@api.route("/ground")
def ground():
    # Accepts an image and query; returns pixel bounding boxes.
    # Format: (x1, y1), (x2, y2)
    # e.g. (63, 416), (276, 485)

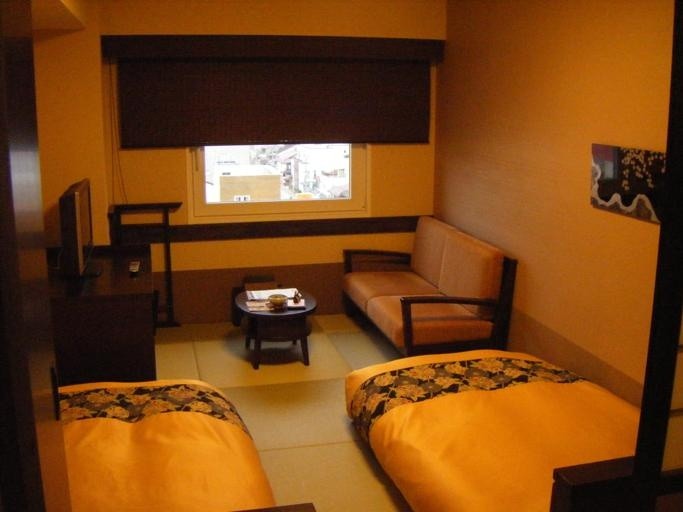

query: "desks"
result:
(55, 244), (159, 383)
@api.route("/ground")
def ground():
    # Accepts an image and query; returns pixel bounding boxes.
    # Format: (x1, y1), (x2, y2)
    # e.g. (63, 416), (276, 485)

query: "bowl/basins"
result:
(268, 294), (288, 308)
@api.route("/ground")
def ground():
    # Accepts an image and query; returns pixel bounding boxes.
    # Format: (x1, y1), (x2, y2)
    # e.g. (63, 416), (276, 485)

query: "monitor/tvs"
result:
(59, 178), (102, 290)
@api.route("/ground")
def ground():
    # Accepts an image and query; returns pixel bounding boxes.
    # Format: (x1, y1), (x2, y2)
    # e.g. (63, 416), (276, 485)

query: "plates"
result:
(286, 297), (307, 309)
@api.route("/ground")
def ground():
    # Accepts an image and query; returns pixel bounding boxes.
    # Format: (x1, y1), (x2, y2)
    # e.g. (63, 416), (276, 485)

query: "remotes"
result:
(128, 260), (141, 272)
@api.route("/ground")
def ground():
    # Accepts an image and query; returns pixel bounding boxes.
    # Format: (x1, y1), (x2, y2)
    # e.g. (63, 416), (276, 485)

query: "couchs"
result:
(340, 211), (517, 354)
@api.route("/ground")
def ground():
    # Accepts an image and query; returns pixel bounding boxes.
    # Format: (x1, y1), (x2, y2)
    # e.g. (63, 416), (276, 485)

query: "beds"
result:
(54, 375), (316, 511)
(343, 346), (637, 511)
